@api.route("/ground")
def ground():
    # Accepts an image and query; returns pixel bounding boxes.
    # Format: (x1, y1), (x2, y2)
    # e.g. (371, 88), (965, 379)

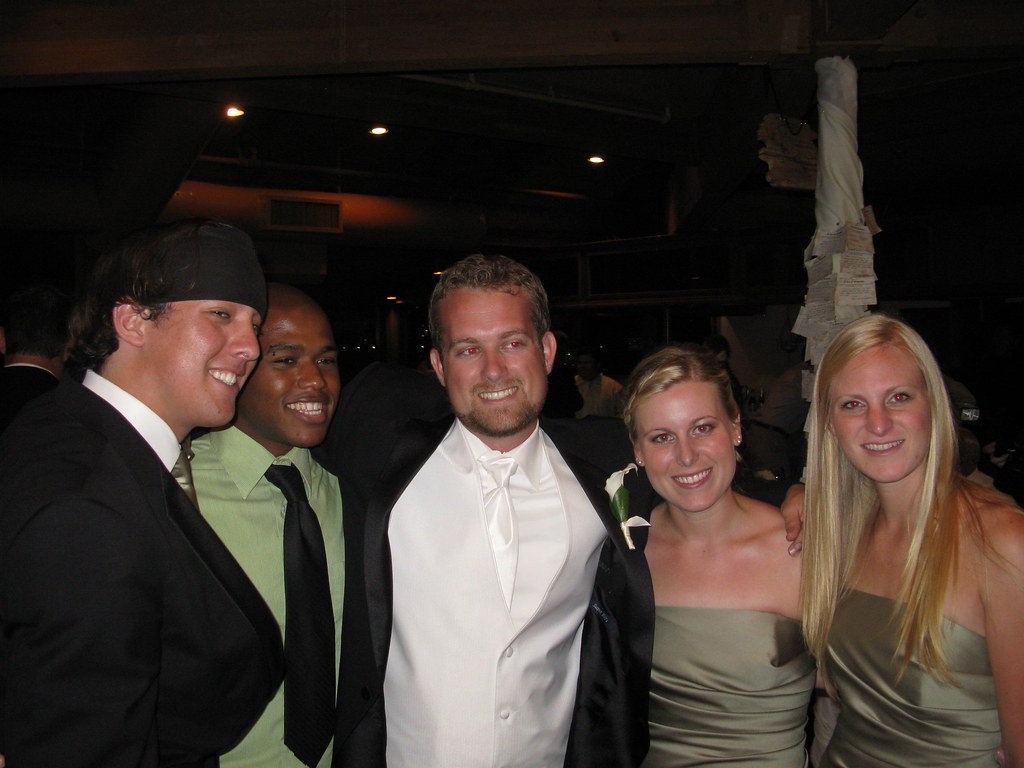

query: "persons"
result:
(189, 283), (345, 768)
(0, 244), (86, 435)
(548, 330), (584, 418)
(960, 424), (1024, 508)
(316, 254), (804, 768)
(803, 313), (1024, 768)
(622, 345), (825, 768)
(0, 222), (286, 768)
(571, 349), (621, 419)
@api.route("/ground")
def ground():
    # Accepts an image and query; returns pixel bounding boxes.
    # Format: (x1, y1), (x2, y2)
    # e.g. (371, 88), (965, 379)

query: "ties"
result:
(480, 457), (519, 607)
(268, 464), (340, 767)
